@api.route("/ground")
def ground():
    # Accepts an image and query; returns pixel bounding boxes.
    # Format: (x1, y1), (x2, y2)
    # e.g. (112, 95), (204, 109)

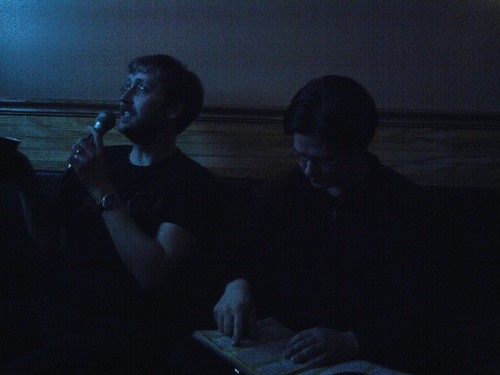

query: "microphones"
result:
(63, 111), (116, 176)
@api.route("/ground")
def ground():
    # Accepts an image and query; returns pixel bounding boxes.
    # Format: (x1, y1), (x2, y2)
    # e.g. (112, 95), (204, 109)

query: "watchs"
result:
(97, 193), (121, 217)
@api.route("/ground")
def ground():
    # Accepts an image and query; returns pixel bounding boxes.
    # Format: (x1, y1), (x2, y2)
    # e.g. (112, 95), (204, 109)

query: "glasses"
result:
(290, 144), (358, 165)
(120, 84), (168, 102)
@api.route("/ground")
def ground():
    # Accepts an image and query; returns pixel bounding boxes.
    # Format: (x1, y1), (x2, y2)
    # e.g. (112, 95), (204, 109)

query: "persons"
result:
(194, 76), (435, 375)
(0, 55), (220, 375)
